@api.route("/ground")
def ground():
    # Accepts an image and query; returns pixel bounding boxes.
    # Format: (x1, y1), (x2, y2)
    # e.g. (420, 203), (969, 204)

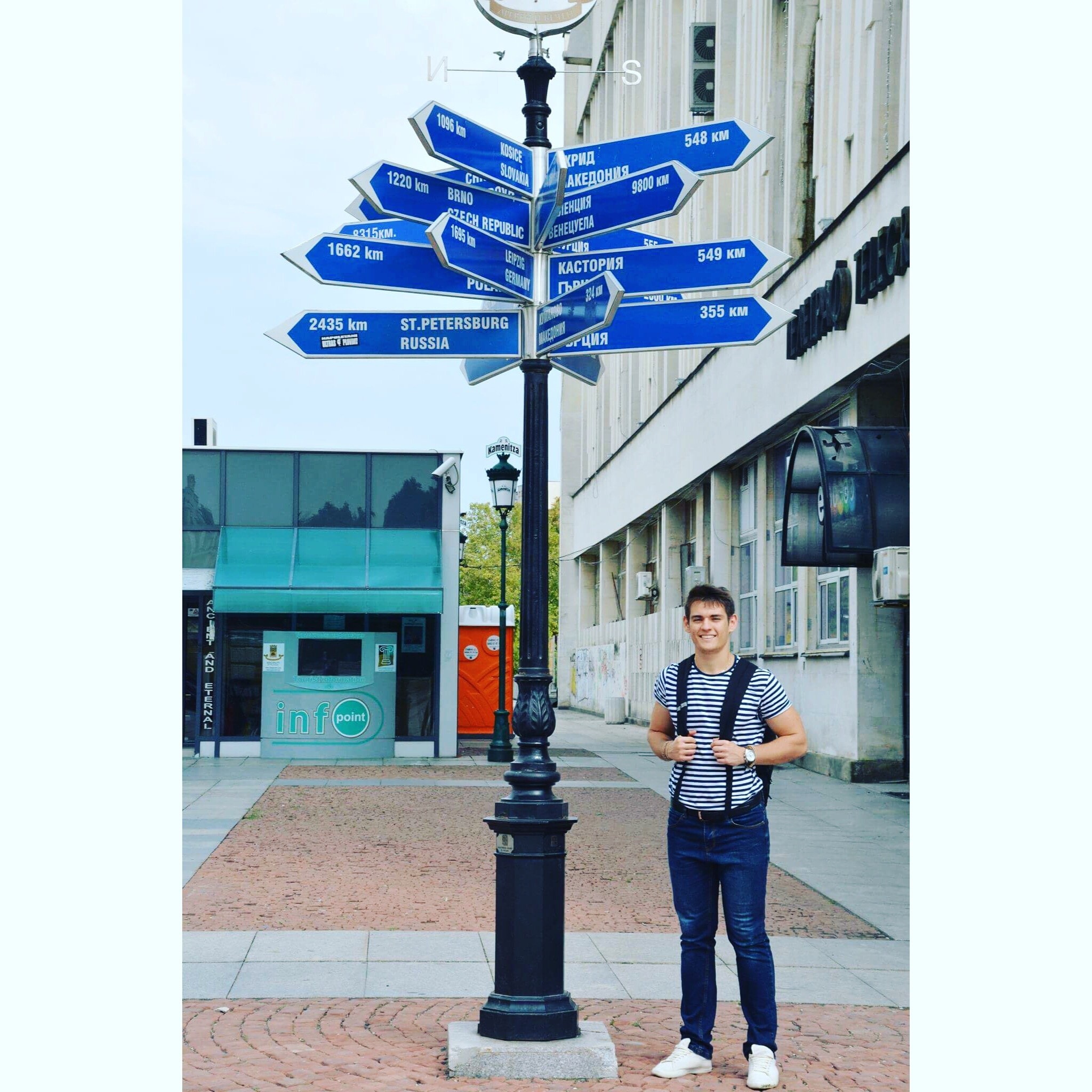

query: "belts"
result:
(670, 792), (762, 822)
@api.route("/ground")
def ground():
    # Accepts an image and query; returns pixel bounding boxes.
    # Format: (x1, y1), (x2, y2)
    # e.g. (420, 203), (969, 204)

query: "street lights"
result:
(485, 437), (521, 762)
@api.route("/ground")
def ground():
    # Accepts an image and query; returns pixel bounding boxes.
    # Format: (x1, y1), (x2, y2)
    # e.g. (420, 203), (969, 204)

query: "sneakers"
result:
(651, 1038), (712, 1079)
(747, 1044), (778, 1088)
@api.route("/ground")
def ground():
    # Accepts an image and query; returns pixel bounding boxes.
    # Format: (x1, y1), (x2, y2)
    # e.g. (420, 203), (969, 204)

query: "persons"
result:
(647, 583), (810, 1091)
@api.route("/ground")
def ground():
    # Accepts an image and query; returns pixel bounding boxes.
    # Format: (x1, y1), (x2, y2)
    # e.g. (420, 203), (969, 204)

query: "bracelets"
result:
(748, 745), (757, 767)
(662, 740), (673, 761)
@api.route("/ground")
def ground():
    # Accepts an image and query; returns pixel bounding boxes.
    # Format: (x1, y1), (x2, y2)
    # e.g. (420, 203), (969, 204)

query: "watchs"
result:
(743, 746), (755, 766)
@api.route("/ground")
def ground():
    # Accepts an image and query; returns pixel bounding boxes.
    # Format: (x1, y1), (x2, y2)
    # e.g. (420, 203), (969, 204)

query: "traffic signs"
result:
(263, 100), (532, 385)
(534, 117), (798, 387)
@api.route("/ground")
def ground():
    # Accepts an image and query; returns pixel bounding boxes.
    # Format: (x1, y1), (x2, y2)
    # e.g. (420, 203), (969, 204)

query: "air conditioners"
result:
(636, 572), (652, 598)
(872, 546), (910, 601)
(688, 22), (716, 112)
(685, 567), (707, 600)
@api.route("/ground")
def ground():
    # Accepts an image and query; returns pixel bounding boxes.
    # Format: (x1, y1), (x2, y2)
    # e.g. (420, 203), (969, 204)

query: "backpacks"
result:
(673, 654), (776, 807)
